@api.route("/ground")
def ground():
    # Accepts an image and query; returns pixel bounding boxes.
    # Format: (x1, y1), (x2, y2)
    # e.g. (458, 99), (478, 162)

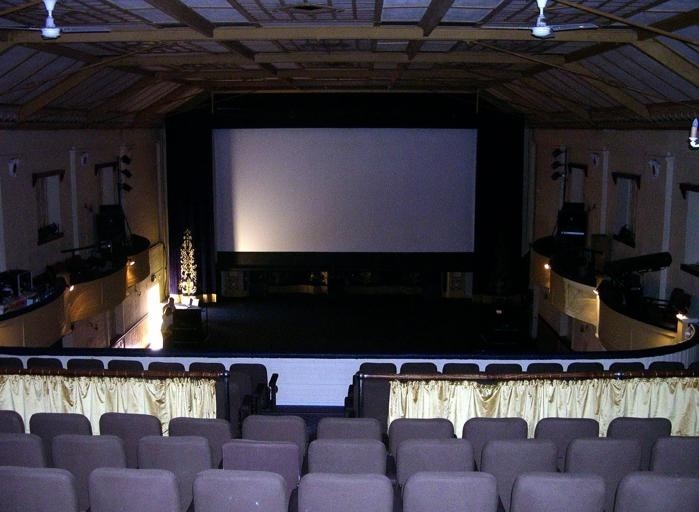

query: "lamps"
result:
(550, 144), (588, 183)
(688, 118), (699, 148)
(94, 156), (136, 194)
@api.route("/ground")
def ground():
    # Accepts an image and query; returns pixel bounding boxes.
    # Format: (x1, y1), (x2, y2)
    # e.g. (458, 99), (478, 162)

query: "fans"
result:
(480, 0), (599, 38)
(0, 0), (154, 41)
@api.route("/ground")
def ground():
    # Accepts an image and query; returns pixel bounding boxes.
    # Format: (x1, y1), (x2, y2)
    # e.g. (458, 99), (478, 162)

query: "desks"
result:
(530, 230), (698, 329)
(1, 231), (149, 315)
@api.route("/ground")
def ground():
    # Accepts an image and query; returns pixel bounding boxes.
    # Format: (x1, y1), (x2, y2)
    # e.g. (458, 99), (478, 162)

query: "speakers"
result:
(556, 200), (588, 254)
(0, 269), (32, 294)
(96, 203), (125, 238)
